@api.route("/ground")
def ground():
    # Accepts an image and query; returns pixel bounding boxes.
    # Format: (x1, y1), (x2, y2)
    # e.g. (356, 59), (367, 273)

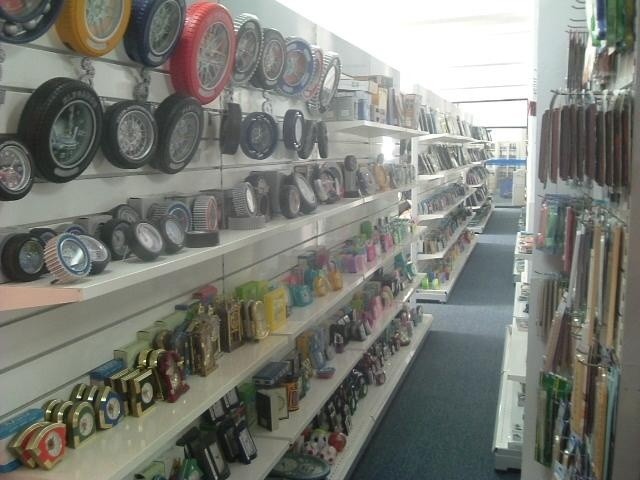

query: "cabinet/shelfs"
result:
(379, 102), (496, 305)
(0, 0), (434, 480)
